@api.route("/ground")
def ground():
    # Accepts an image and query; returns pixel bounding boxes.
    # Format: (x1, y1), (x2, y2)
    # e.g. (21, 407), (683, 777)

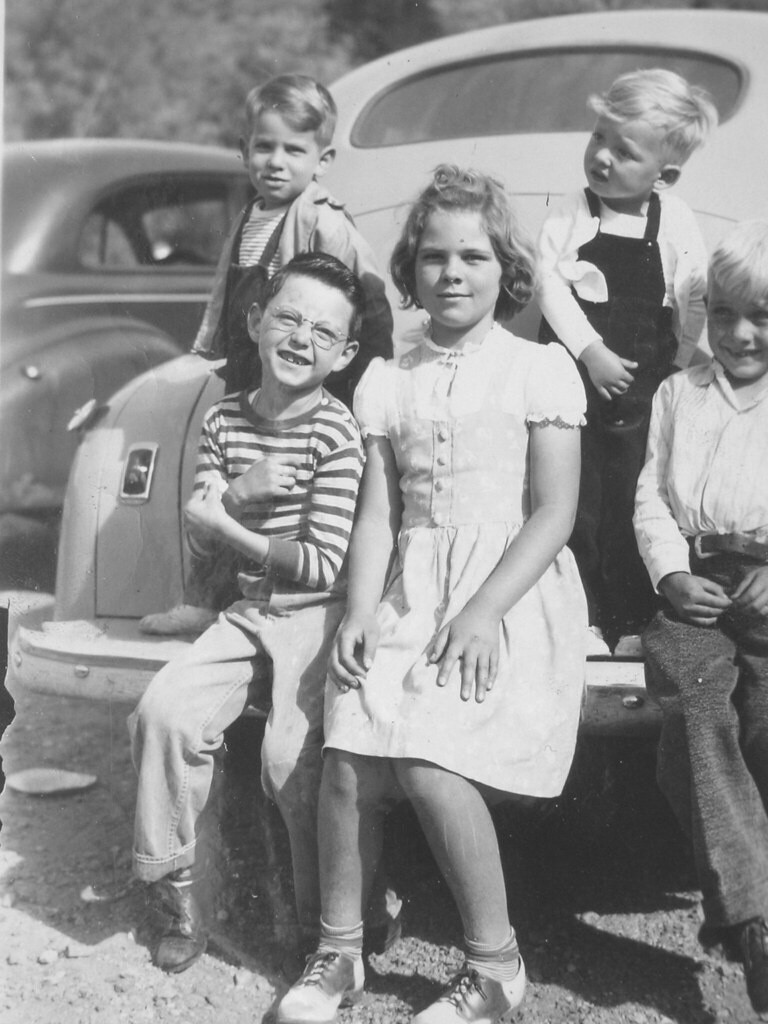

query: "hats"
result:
(162, 225), (222, 264)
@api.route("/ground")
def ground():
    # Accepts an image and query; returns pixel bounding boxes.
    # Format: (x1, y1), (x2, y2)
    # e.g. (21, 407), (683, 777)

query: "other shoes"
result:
(731, 919), (768, 1013)
(132, 873), (218, 975)
(137, 602), (219, 634)
(613, 634), (644, 658)
(282, 935), (320, 986)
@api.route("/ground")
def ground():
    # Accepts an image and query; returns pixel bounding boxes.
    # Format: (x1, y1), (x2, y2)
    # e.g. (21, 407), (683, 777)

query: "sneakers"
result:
(411, 952), (526, 1024)
(276, 949), (365, 1024)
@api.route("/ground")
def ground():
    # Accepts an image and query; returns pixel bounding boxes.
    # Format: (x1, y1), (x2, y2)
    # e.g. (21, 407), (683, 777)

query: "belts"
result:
(686, 532), (768, 563)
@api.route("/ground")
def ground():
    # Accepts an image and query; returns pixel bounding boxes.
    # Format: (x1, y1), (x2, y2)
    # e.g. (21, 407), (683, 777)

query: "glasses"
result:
(262, 302), (353, 350)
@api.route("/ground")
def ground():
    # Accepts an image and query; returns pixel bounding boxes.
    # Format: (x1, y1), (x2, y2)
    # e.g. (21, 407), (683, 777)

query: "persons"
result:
(631, 226), (768, 1014)
(136, 72), (384, 639)
(531, 66), (719, 663)
(125, 251), (370, 971)
(274, 160), (590, 1024)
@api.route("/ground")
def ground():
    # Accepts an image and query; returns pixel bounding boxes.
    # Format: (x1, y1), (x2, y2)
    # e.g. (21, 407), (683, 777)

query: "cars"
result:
(316, 10), (768, 366)
(0, 140), (257, 582)
